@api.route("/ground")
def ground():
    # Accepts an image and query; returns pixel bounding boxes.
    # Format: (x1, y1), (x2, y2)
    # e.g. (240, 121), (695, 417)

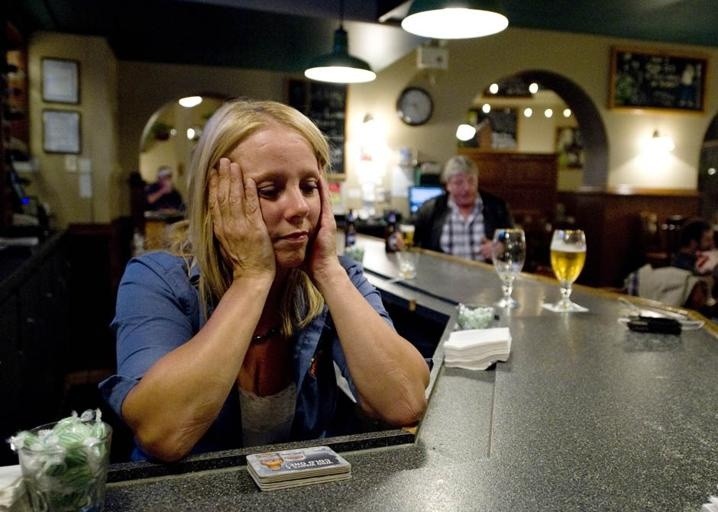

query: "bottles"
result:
(385, 211), (397, 253)
(343, 209), (356, 247)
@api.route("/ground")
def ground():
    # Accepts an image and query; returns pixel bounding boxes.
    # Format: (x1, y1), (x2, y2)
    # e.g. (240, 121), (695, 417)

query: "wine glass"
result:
(548, 228), (588, 313)
(492, 228), (525, 311)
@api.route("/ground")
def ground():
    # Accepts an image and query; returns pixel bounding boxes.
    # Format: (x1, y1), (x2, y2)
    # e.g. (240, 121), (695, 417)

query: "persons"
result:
(97, 96), (431, 464)
(144, 164), (183, 212)
(695, 248), (718, 286)
(621, 220), (717, 313)
(394, 155), (516, 266)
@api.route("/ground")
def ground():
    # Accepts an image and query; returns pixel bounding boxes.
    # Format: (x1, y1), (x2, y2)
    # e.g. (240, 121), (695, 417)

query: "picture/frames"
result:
(39, 56), (80, 104)
(40, 108), (82, 154)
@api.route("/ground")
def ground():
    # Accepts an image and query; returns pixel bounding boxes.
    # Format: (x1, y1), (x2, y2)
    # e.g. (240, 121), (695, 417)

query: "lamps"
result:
(304, 17), (377, 84)
(400, 0), (509, 40)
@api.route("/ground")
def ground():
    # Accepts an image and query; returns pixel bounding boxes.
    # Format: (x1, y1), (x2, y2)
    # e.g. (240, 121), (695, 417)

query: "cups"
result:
(19, 420), (114, 512)
(344, 246), (365, 263)
(396, 251), (420, 279)
(455, 304), (495, 331)
(398, 225), (416, 251)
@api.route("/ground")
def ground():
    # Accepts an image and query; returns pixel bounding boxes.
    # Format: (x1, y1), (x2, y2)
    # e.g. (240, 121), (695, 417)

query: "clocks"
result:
(397, 87), (433, 126)
